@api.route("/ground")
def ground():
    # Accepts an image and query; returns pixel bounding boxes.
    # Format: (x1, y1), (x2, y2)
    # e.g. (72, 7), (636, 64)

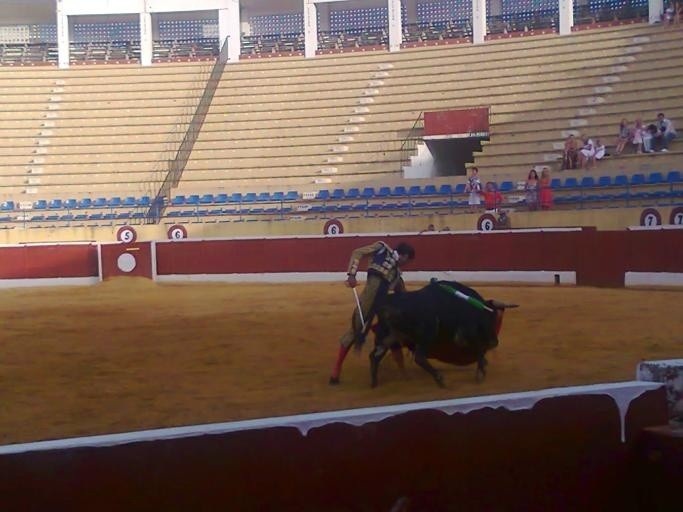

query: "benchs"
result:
(0, 20), (682, 194)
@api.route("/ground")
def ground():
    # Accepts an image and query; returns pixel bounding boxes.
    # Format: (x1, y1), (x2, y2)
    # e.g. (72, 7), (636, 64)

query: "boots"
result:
(329, 345), (348, 386)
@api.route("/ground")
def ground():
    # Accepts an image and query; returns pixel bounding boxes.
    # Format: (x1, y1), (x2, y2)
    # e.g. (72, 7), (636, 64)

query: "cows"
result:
(352, 279), (521, 389)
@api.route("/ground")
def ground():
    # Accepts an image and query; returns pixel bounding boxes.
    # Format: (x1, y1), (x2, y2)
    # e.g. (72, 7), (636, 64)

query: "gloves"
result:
(348, 275), (357, 288)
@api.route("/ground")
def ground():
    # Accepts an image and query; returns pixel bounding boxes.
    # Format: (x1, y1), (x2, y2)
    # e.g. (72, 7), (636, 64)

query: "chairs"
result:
(0, 172), (682, 222)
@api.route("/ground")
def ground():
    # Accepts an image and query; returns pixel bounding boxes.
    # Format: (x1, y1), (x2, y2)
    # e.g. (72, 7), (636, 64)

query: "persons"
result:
(524, 113), (678, 211)
(468, 178), (481, 209)
(474, 182), (503, 216)
(499, 210), (512, 228)
(664, 0), (683, 25)
(419, 224), (434, 235)
(330, 241), (415, 385)
(465, 168), (479, 190)
